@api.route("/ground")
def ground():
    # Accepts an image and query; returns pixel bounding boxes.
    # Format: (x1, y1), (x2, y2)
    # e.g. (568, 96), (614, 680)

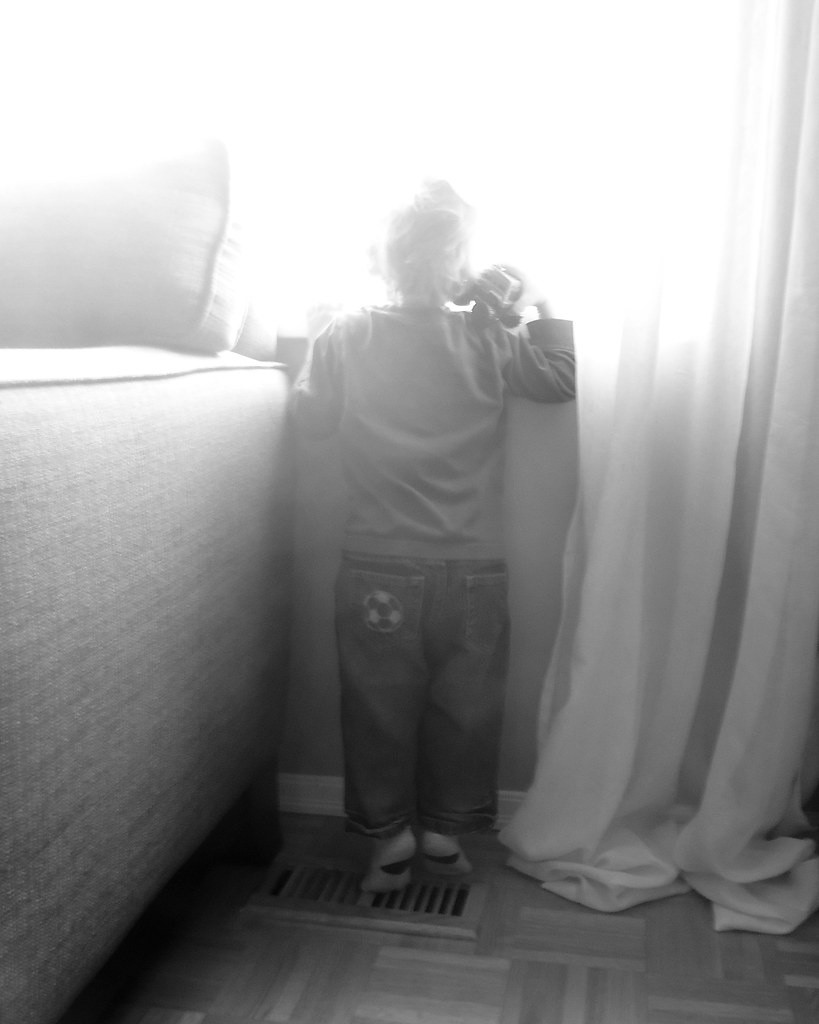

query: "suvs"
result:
(452, 263), (522, 328)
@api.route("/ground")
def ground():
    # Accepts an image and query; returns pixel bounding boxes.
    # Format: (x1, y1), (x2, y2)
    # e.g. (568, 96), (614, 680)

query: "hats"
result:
(387, 181), (476, 270)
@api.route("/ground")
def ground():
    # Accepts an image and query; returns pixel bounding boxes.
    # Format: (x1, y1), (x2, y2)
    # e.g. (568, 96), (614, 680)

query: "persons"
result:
(279, 177), (583, 899)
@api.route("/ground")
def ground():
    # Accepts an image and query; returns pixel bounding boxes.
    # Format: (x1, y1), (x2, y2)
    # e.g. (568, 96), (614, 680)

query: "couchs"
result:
(0, 151), (291, 1021)
